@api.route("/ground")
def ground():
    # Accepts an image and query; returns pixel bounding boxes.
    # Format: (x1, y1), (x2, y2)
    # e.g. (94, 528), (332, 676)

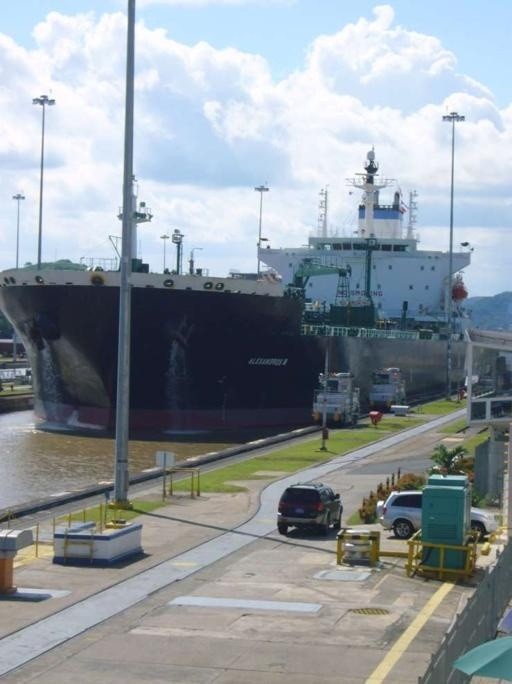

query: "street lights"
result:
(441, 110), (466, 399)
(251, 185), (268, 273)
(160, 235), (169, 271)
(190, 247), (204, 259)
(10, 193), (27, 268)
(28, 92), (55, 269)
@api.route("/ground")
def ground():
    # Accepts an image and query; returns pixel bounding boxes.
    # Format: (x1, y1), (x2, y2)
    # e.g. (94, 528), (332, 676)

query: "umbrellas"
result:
(448, 634), (510, 683)
(492, 605), (511, 634)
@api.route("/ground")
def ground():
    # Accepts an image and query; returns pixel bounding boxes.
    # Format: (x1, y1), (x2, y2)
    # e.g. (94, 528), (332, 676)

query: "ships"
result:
(1, 143), (505, 436)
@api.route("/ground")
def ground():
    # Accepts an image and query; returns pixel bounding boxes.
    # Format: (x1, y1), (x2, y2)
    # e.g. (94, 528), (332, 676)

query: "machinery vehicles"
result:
(367, 367), (408, 411)
(312, 371), (361, 427)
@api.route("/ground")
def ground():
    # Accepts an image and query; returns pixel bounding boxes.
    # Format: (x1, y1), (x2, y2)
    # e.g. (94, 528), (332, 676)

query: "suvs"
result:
(276, 480), (343, 536)
(376, 488), (500, 540)
